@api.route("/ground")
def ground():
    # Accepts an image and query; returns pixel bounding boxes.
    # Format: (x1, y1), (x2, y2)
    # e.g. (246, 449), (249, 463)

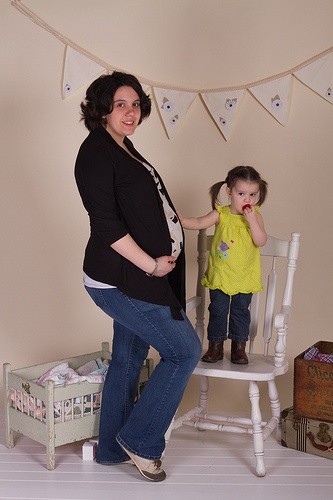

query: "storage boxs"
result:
(280, 341), (333, 461)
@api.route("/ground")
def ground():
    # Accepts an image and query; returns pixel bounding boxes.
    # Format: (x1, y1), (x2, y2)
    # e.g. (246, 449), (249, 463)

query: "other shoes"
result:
(123, 447), (167, 482)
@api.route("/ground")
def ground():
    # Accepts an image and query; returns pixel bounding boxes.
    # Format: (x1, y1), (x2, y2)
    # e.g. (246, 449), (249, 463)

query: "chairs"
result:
(165, 227), (306, 477)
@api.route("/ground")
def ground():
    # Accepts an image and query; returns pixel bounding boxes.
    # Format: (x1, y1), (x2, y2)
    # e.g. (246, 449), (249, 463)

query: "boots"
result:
(200, 340), (224, 363)
(231, 339), (249, 364)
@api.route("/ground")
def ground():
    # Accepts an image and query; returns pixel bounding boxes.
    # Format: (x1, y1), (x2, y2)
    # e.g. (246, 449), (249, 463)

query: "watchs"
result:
(146, 258), (158, 278)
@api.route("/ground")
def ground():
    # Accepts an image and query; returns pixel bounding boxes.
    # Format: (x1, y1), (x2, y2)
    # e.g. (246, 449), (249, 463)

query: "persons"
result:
(74, 70), (204, 482)
(176, 166), (268, 363)
